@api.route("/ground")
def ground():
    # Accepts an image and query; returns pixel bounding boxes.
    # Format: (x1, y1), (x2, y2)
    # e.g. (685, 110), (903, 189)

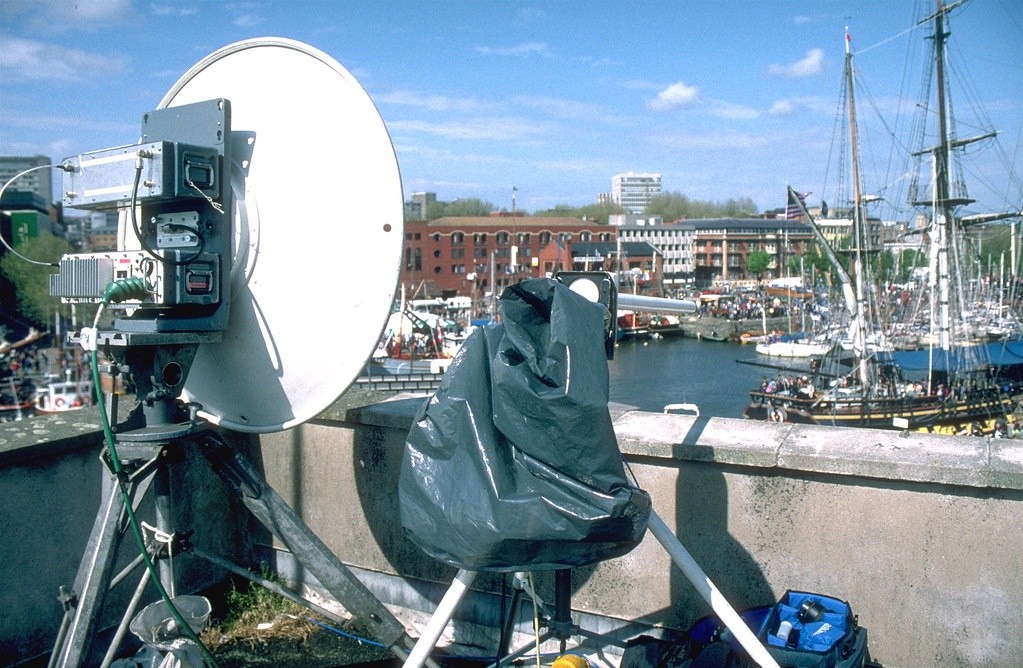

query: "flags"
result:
(436, 322), (442, 344)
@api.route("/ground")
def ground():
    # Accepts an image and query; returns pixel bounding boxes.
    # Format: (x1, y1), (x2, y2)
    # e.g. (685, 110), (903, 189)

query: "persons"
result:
(875, 379), (927, 398)
(839, 372), (853, 388)
(389, 328), (426, 353)
(761, 373), (815, 409)
(937, 383), (946, 402)
(700, 299), (761, 318)
(9, 347), (48, 376)
(956, 383), (965, 401)
(65, 351), (73, 362)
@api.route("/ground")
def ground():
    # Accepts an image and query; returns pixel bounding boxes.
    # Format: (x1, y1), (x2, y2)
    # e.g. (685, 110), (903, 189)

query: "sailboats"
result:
(731, 0), (1023, 440)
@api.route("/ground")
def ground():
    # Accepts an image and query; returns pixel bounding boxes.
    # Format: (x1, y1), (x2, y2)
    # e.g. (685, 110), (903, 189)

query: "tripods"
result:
(402, 510), (784, 668)
(48, 329), (450, 668)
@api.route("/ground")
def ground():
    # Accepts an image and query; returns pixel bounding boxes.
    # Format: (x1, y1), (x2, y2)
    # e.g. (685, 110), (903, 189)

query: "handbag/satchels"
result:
(686, 590), (870, 668)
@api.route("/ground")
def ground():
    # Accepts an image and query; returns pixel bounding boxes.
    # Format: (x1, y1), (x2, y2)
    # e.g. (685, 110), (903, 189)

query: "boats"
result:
(604, 210), (1023, 360)
(33, 381), (94, 416)
(351, 251), (494, 397)
